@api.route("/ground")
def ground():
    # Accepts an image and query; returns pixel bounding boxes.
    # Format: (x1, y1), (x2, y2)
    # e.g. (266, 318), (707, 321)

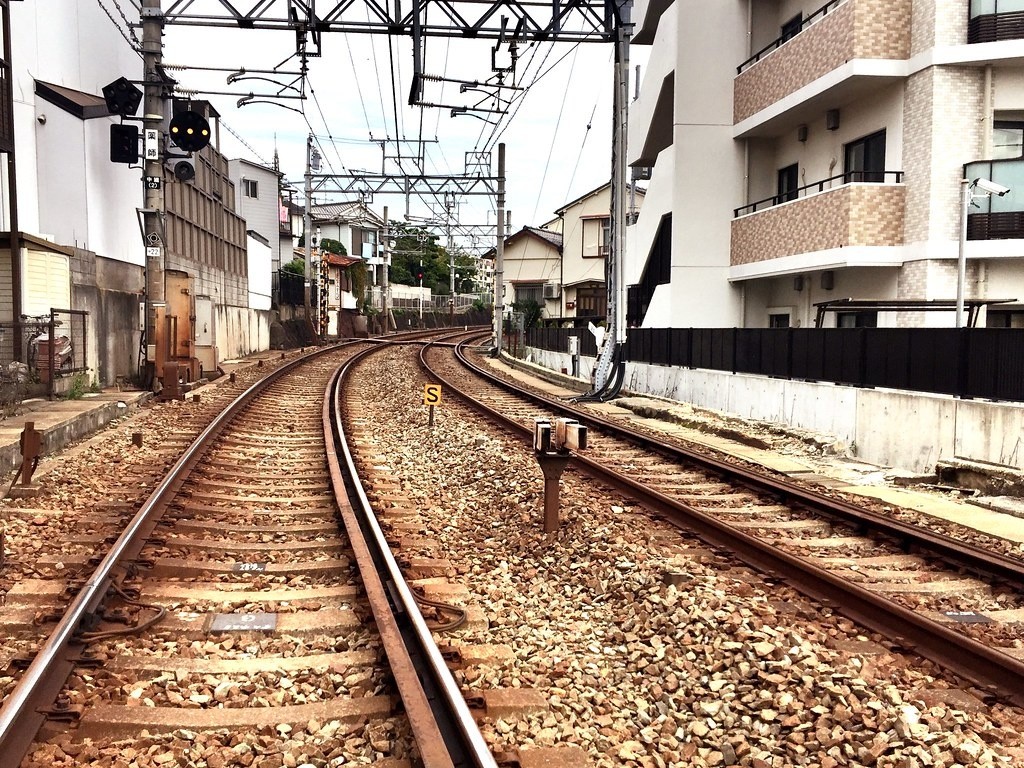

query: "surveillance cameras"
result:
(974, 178), (1010, 197)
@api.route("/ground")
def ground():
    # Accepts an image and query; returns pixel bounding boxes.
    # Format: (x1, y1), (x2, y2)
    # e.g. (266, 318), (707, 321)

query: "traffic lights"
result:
(418, 273), (423, 278)
